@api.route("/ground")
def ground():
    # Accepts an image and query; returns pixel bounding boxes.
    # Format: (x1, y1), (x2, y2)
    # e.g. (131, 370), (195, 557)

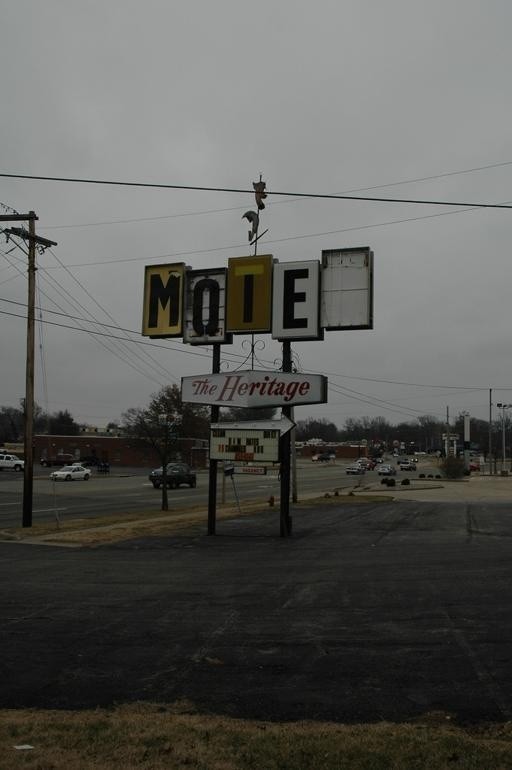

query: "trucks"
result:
(40, 454), (80, 467)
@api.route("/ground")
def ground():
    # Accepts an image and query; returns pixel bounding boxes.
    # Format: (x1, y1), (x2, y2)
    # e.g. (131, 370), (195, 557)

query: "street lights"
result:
(497, 402), (512, 475)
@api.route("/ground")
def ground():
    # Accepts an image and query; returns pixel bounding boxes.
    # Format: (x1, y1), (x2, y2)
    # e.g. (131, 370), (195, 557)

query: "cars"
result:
(149, 463), (196, 489)
(345, 453), (418, 476)
(0, 454), (24, 471)
(312, 454), (330, 462)
(469, 462), (480, 471)
(49, 466), (92, 481)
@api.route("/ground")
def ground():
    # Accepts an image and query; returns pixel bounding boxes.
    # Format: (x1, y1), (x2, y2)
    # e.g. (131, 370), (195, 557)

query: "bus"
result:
(0, 443), (24, 454)
